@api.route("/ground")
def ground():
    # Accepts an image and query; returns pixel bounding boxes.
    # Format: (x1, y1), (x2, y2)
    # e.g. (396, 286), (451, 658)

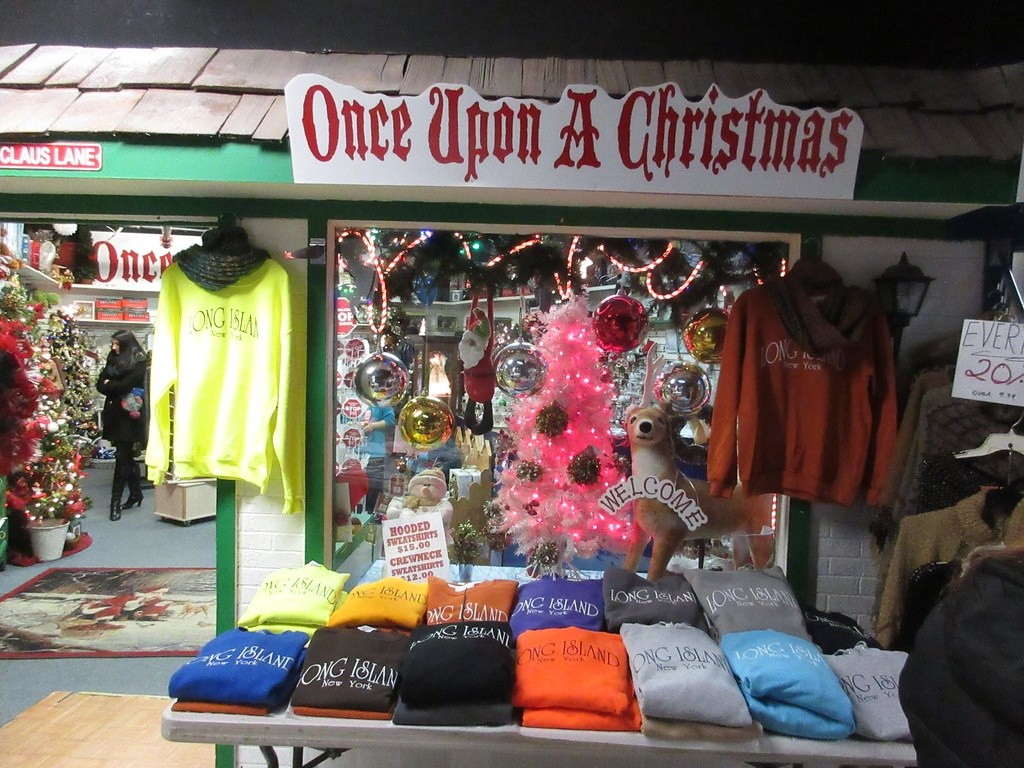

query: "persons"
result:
(96, 330), (151, 521)
(407, 424), (462, 491)
(340, 362), (397, 514)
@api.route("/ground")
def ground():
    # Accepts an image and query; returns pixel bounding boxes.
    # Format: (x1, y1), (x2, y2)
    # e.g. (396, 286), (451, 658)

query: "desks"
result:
(161, 697), (920, 768)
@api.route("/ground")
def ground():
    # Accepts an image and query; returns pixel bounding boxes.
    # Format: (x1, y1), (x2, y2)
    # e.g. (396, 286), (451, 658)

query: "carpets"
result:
(0, 566), (218, 660)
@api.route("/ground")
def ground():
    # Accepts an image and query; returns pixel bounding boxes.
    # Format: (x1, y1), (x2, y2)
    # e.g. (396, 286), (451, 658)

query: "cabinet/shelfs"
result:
(15, 256), (164, 327)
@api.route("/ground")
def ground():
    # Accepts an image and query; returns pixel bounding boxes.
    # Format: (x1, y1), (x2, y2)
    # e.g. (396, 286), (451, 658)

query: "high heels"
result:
(121, 493), (143, 509)
(110, 499), (122, 521)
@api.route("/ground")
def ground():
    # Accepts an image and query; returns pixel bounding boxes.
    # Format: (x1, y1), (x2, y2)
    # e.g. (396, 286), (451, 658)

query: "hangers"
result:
(900, 286), (1024, 566)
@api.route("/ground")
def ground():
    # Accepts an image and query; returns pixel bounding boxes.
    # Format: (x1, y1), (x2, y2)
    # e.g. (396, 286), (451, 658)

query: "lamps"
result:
(870, 250), (938, 369)
